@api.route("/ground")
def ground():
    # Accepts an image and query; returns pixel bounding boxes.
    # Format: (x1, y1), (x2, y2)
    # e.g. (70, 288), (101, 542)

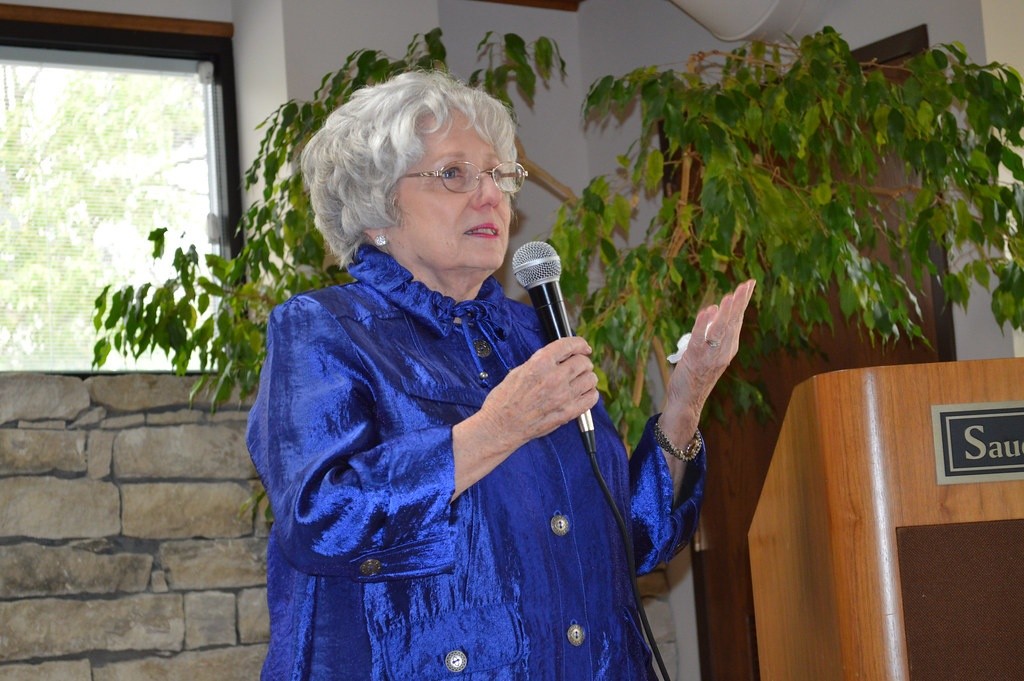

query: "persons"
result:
(248, 71), (757, 681)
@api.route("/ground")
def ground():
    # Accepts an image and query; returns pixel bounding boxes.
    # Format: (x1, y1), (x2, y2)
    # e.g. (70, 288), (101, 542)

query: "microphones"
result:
(512, 242), (597, 455)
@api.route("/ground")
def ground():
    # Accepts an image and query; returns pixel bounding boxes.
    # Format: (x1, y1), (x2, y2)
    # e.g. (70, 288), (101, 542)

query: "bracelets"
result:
(656, 423), (702, 462)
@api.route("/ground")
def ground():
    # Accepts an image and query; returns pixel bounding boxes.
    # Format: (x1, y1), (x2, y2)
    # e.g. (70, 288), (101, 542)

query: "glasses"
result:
(400, 160), (528, 194)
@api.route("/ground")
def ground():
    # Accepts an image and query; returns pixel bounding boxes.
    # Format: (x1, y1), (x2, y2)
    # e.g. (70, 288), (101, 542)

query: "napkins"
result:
(666, 321), (712, 363)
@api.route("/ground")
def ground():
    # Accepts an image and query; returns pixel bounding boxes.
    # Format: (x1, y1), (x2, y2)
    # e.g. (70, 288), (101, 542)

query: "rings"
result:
(706, 337), (721, 347)
